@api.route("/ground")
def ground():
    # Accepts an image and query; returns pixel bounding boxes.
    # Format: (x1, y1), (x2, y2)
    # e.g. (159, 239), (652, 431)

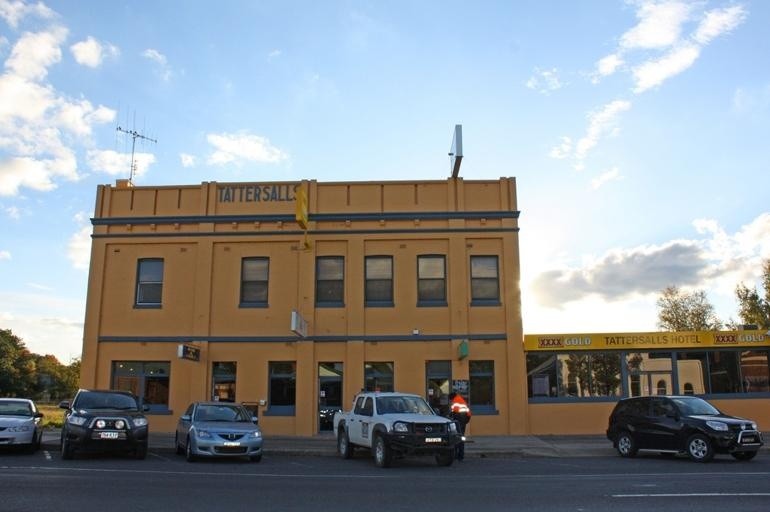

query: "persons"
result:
(448, 392), (472, 460)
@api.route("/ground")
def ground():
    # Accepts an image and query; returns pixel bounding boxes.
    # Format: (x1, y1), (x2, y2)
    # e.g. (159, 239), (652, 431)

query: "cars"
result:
(0, 396), (47, 453)
(173, 400), (265, 464)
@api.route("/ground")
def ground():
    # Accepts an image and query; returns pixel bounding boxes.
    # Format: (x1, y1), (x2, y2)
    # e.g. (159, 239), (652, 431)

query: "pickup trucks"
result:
(331, 391), (465, 469)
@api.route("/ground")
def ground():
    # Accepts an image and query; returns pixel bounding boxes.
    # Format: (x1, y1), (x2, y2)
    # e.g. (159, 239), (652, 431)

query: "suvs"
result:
(57, 388), (150, 461)
(605, 393), (765, 463)
